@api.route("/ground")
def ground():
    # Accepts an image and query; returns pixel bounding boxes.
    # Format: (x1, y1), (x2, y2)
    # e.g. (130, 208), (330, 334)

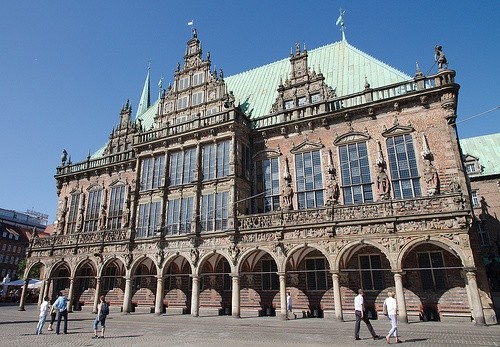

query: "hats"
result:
(58, 292), (63, 296)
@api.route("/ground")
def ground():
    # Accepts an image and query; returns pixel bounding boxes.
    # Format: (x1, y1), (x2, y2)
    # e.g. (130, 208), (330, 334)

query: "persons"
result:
(286, 291), (297, 319)
(1, 286), (38, 299)
(48, 300), (57, 331)
(479, 196), (491, 215)
(35, 295), (50, 334)
(3, 273), (11, 292)
(91, 295), (107, 338)
(383, 291), (402, 344)
(53, 292), (69, 335)
(353, 289), (380, 340)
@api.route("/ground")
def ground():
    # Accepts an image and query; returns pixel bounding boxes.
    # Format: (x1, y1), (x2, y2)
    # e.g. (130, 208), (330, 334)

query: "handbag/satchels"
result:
(382, 301), (387, 315)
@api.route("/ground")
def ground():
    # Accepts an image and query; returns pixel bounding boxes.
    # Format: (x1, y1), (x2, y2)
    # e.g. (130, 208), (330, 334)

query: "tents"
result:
(3, 278), (47, 288)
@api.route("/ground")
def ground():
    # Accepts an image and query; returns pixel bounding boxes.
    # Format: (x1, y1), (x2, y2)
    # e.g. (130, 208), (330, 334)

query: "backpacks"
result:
(102, 303), (109, 315)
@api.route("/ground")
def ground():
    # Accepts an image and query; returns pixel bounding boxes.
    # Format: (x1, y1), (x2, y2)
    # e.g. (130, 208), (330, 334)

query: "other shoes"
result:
(374, 335), (383, 339)
(396, 340), (401, 343)
(63, 332), (69, 334)
(35, 334), (37, 335)
(356, 338), (361, 340)
(386, 336), (390, 344)
(92, 335), (97, 338)
(48, 328), (52, 331)
(295, 315), (297, 319)
(39, 333), (43, 334)
(56, 333), (59, 335)
(98, 336), (104, 338)
